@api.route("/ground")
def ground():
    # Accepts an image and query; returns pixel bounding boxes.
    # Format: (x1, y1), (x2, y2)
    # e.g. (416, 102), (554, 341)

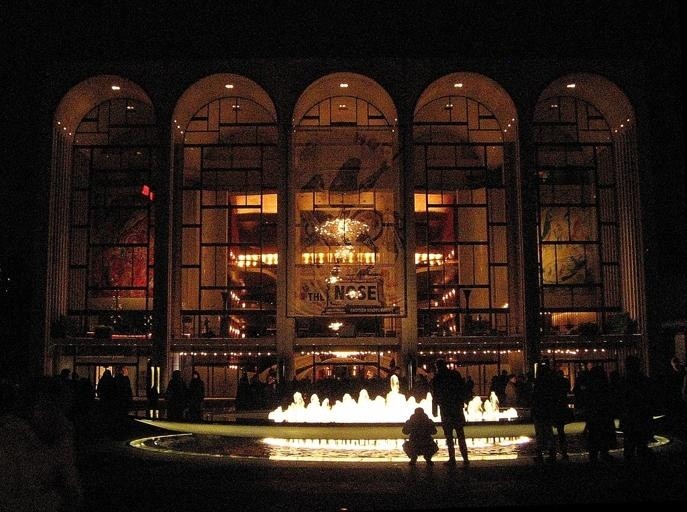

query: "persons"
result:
(402, 408), (439, 466)
(430, 360), (471, 465)
(2, 367), (204, 511)
(491, 354), (687, 473)
(238, 372), (475, 413)
